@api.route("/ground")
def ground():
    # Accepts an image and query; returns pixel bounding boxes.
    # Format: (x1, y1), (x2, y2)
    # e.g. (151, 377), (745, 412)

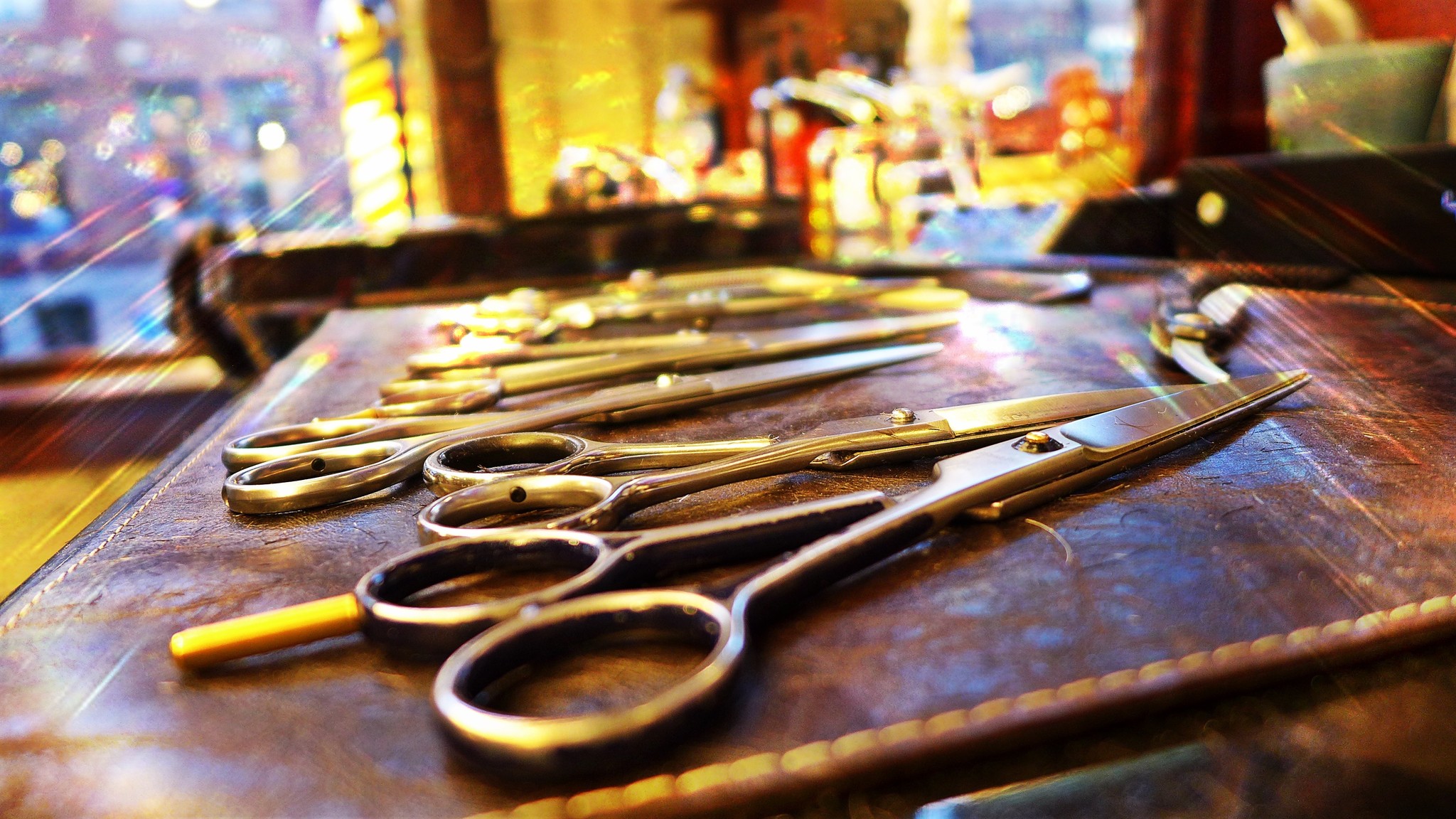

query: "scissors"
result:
(170, 267), (1316, 783)
